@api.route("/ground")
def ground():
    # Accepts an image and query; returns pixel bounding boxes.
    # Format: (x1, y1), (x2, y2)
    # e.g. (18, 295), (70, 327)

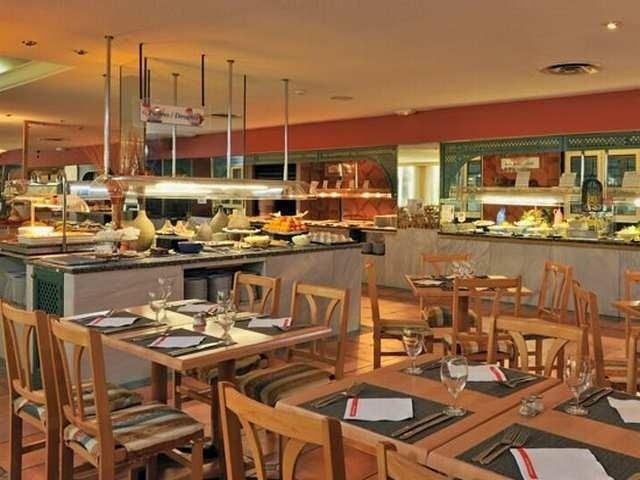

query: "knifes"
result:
(390, 412), (454, 440)
(569, 386), (613, 407)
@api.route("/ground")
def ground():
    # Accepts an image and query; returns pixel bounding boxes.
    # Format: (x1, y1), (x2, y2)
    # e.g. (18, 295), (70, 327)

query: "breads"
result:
(21, 218), (105, 233)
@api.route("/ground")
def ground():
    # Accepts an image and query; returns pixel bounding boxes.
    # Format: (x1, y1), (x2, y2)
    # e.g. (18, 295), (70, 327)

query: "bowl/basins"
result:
(177, 242), (203, 253)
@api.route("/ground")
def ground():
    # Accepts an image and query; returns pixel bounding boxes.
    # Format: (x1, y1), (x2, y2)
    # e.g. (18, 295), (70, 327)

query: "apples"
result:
(263, 216), (306, 233)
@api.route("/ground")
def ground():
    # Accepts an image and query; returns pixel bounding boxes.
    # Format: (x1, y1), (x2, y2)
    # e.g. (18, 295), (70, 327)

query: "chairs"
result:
(486, 318), (586, 386)
(361, 258), (433, 372)
(215, 381), (379, 480)
(374, 441), (445, 480)
(623, 268), (639, 356)
(570, 278), (640, 392)
(172, 273), (279, 434)
(418, 252), (480, 329)
(445, 278), (520, 362)
(520, 260), (571, 376)
(232, 283), (347, 405)
(1, 301), (141, 479)
(46, 312), (208, 478)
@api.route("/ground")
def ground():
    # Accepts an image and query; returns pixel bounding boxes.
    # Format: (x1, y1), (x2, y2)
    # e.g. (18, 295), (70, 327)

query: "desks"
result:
(428, 380), (638, 480)
(609, 299), (640, 320)
(407, 270), (523, 331)
(61, 299), (329, 475)
(280, 351), (562, 456)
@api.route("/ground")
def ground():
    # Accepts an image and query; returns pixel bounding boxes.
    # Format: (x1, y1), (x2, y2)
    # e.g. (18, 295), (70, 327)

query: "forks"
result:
(470, 426), (530, 466)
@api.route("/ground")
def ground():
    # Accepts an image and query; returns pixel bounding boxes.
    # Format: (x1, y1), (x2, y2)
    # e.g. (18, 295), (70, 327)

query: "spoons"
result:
(310, 381), (366, 409)
(497, 375), (538, 389)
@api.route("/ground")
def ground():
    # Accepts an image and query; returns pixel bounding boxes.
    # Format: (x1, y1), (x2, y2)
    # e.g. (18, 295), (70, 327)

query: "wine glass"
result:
(563, 355), (590, 416)
(440, 355), (468, 416)
(147, 273), (177, 326)
(216, 289), (236, 346)
(402, 326), (424, 375)
